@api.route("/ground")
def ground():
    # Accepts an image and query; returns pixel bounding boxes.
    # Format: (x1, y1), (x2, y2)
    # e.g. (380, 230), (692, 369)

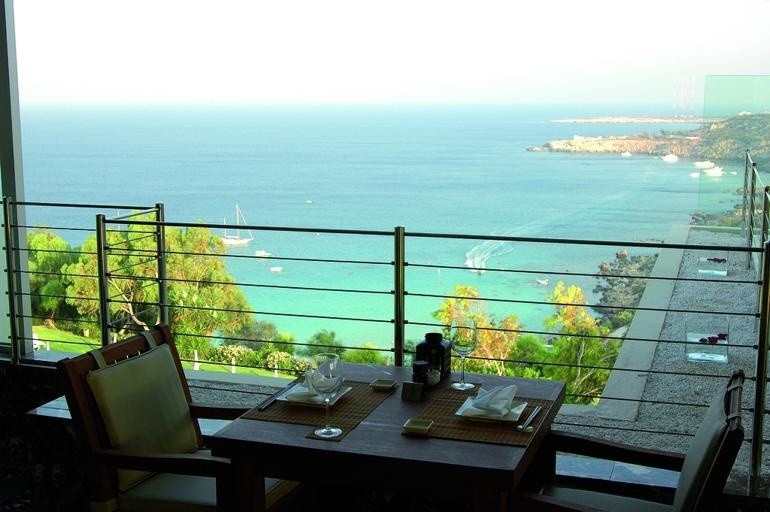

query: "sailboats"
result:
(214, 204), (257, 246)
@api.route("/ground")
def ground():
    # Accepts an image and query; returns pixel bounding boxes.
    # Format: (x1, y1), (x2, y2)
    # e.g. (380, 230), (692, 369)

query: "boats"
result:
(270, 265), (282, 272)
(663, 154), (679, 163)
(690, 159), (727, 180)
(473, 255), (481, 271)
(254, 248), (273, 258)
(622, 152), (631, 158)
(536, 278), (549, 286)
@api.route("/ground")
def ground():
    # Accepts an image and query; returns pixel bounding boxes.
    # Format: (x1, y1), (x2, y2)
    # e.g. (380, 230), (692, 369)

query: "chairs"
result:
(56, 325), (304, 511)
(529, 367), (748, 512)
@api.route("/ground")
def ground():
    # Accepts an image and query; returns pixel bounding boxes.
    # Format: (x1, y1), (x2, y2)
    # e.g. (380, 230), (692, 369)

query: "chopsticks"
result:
(257, 381), (296, 411)
(520, 405), (543, 430)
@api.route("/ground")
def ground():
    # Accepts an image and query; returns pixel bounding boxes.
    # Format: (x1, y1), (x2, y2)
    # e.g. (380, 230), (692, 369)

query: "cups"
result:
(695, 238), (730, 277)
(682, 313), (731, 366)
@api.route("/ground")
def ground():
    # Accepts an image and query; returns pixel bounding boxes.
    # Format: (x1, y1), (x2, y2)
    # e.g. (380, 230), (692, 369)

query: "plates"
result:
(403, 417), (434, 434)
(276, 381), (353, 409)
(368, 378), (398, 389)
(453, 396), (528, 423)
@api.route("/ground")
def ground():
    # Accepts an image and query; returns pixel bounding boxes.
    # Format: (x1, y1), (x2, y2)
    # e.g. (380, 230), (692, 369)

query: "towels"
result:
(284, 378), (335, 403)
(462, 382), (519, 419)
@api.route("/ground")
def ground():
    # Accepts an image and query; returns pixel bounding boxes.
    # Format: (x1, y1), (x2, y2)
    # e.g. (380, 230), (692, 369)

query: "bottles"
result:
(410, 360), (429, 385)
(417, 331), (452, 381)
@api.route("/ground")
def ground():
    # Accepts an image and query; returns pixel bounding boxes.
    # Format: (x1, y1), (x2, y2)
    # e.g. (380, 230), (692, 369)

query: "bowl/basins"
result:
(410, 370), (440, 386)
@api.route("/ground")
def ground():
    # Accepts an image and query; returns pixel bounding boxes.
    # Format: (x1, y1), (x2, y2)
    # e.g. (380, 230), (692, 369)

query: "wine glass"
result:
(449, 317), (476, 391)
(311, 351), (344, 439)
